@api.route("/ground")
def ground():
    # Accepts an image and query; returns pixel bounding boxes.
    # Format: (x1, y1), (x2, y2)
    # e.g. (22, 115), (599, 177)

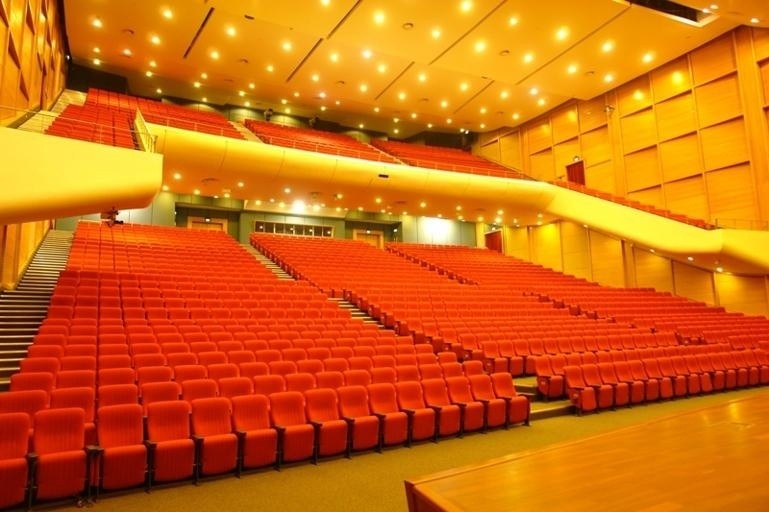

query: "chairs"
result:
(549, 177), (718, 233)
(39, 87), (538, 182)
(1, 219), (769, 509)
